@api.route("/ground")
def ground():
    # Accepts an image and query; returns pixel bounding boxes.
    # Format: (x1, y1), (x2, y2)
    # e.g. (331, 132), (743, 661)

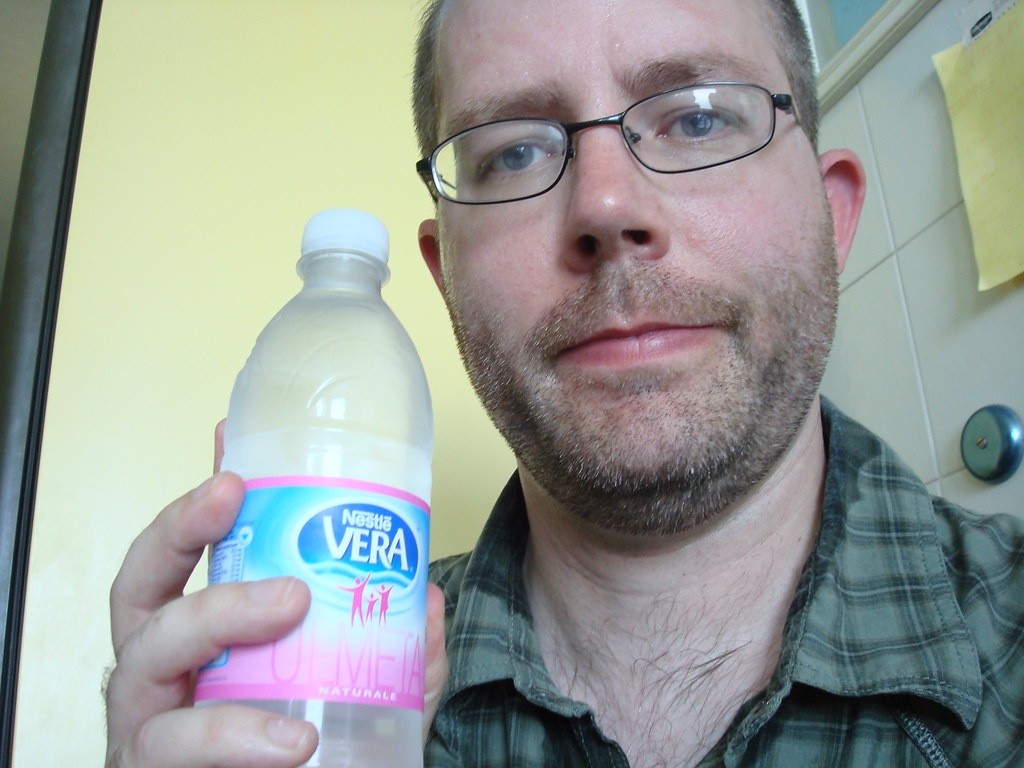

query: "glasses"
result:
(416, 80), (803, 206)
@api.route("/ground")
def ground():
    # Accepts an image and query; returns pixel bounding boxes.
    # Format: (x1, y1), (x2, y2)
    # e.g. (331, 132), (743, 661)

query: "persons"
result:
(106, 0), (1024, 768)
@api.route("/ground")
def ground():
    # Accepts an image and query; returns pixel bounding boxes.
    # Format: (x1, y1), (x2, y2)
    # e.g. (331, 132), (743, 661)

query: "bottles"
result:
(193, 206), (435, 768)
